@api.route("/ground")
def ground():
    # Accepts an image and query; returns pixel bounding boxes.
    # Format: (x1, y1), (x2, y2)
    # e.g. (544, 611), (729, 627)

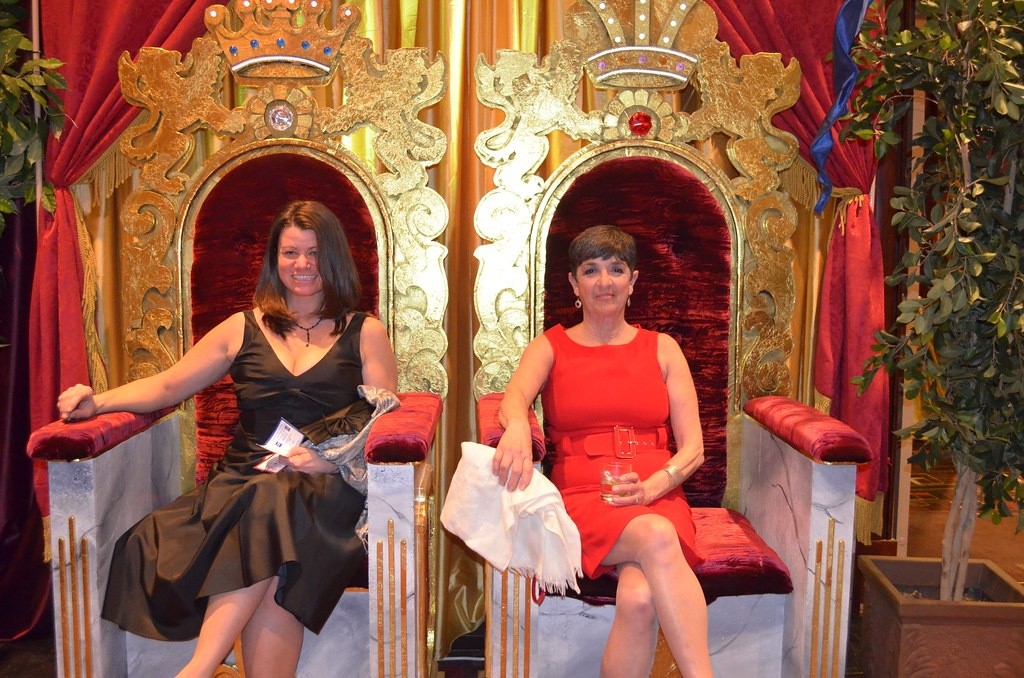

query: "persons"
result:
(489, 224), (715, 678)
(56, 200), (401, 676)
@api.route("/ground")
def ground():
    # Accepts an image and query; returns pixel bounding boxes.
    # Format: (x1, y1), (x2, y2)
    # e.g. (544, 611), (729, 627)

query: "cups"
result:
(600, 462), (634, 502)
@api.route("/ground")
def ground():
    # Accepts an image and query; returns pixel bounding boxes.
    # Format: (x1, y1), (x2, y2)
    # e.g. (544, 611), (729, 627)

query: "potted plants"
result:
(824, 0), (1023, 678)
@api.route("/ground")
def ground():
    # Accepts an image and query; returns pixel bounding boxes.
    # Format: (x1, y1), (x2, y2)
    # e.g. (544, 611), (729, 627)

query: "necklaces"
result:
(283, 309), (326, 344)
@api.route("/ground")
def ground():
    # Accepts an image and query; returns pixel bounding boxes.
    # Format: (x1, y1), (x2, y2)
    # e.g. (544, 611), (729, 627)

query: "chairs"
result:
(472, 0), (874, 678)
(25, 0), (452, 678)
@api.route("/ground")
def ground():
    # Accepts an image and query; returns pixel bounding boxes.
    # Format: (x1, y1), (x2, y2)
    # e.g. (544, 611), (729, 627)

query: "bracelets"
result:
(662, 463), (686, 491)
